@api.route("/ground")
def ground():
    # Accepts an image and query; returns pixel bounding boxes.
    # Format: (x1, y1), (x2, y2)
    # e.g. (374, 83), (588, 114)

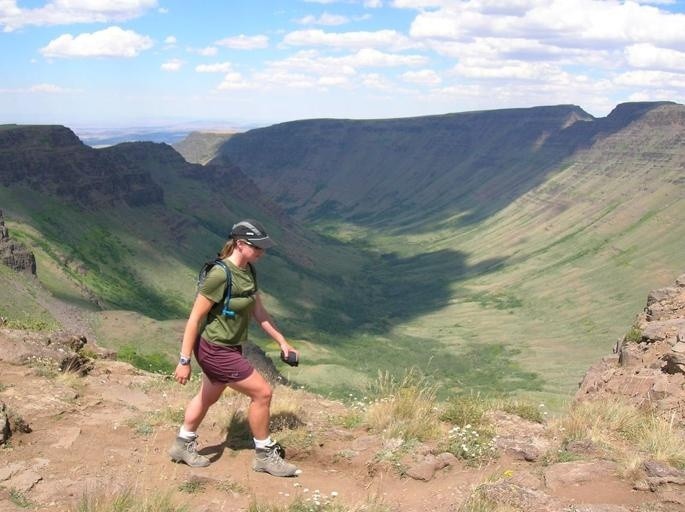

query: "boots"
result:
(253, 441), (297, 477)
(168, 436), (212, 467)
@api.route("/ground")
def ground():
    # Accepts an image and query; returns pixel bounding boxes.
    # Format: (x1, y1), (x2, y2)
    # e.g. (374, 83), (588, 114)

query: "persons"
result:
(166, 218), (301, 478)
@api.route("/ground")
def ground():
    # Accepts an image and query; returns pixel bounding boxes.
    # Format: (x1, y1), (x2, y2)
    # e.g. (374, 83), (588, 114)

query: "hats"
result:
(229, 219), (277, 249)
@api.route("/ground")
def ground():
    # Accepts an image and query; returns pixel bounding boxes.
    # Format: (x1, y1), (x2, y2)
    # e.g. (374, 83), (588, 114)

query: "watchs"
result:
(179, 352), (192, 366)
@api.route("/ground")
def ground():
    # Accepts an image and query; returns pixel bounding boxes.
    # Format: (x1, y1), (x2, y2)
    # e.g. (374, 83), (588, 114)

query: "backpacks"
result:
(197, 259), (256, 307)
